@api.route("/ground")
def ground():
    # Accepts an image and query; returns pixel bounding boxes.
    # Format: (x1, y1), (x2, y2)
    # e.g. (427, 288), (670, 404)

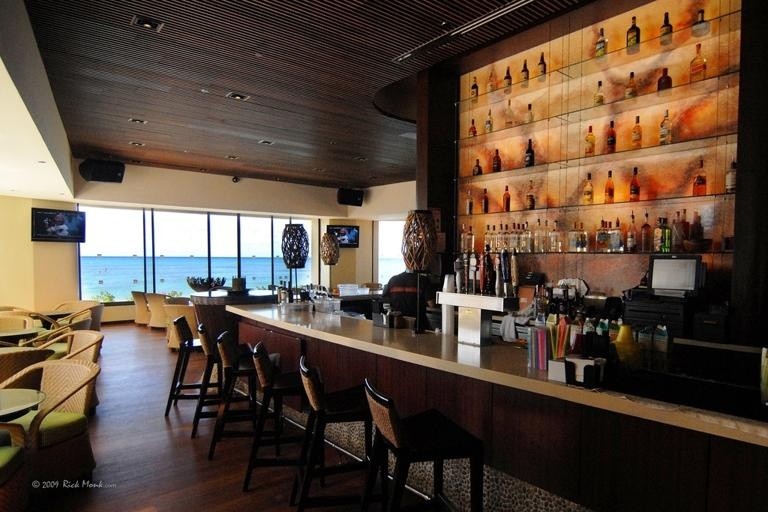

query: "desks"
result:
(0, 388), (45, 425)
(190, 288), (279, 343)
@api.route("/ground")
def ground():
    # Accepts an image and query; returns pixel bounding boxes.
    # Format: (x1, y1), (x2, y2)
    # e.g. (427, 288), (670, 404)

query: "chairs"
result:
(142, 293), (190, 329)
(0, 359), (102, 493)
(241, 341), (327, 493)
(288, 354), (388, 512)
(35, 330), (104, 418)
(207, 331), (282, 461)
(130, 291), (151, 326)
(0, 299), (104, 348)
(190, 322), (256, 440)
(0, 422), (35, 512)
(0, 350), (55, 391)
(268, 283), (382, 301)
(162, 304), (197, 351)
(361, 377), (486, 512)
(164, 315), (225, 418)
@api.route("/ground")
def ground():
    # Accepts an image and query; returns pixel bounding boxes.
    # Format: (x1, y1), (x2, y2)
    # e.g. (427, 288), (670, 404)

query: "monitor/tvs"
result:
(326, 224), (361, 249)
(30, 207), (86, 243)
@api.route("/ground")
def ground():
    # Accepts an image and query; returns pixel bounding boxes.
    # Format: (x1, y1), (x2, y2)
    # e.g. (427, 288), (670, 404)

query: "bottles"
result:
(629, 115), (642, 150)
(472, 158), (482, 176)
(459, 223), (474, 253)
(583, 125), (595, 158)
(465, 190), (473, 215)
(581, 172), (594, 205)
(523, 103), (535, 129)
(467, 118), (477, 142)
(482, 207), (702, 253)
(486, 70), (496, 98)
(519, 58), (528, 88)
(690, 42), (707, 89)
(657, 64), (672, 98)
(484, 108), (494, 136)
(725, 160), (736, 193)
(501, 184), (511, 212)
(524, 138), (534, 165)
(480, 188), (489, 215)
(603, 169), (615, 204)
(658, 11), (673, 45)
(300, 283), (326, 302)
(593, 79), (607, 113)
(625, 15), (640, 55)
(532, 282), (585, 321)
(691, 158), (706, 196)
(535, 52), (546, 83)
(628, 166), (640, 203)
(594, 27), (609, 63)
(524, 182), (536, 209)
(277, 280), (292, 304)
(690, 10), (710, 37)
(660, 107), (672, 146)
(502, 65), (512, 94)
(491, 147), (501, 172)
(469, 76), (478, 104)
(625, 71), (637, 105)
(604, 121), (618, 151)
(504, 99), (513, 131)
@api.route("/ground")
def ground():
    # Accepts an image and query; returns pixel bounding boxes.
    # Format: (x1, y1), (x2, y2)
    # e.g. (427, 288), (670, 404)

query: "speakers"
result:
(77, 157), (126, 183)
(337, 187), (365, 206)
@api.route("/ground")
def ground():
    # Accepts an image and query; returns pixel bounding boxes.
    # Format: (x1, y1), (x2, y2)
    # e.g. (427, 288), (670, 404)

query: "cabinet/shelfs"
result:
(451, 1), (743, 320)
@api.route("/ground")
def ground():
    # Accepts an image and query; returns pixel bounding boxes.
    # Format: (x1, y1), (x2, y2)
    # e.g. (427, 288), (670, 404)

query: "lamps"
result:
(400, 210), (437, 334)
(282, 223), (309, 302)
(320, 233), (340, 291)
